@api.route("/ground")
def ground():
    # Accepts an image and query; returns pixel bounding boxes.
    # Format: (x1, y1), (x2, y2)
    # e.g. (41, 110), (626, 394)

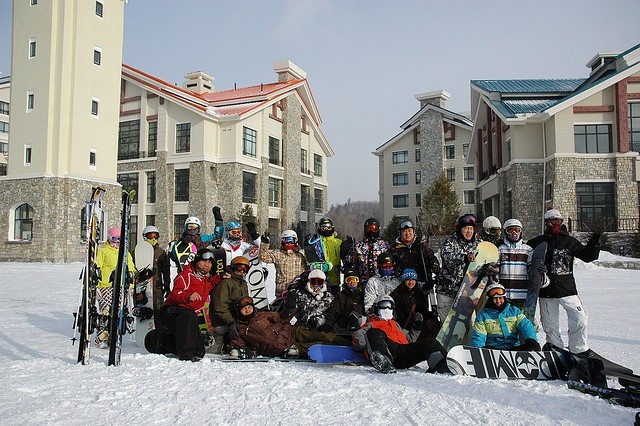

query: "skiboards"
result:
(108, 189), (135, 367)
(77, 186), (105, 365)
(208, 356), (313, 362)
(567, 369), (640, 409)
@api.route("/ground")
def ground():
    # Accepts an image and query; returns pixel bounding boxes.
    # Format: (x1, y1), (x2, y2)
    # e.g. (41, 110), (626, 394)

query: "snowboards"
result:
(304, 234), (332, 294)
(578, 348), (633, 374)
(340, 236), (359, 274)
(525, 242), (548, 324)
(445, 344), (575, 380)
(243, 245), (268, 312)
(542, 342), (608, 388)
(420, 234), (441, 321)
(175, 240), (214, 346)
(209, 247), (228, 327)
(435, 241), (500, 353)
(134, 240), (154, 349)
(307, 344), (368, 364)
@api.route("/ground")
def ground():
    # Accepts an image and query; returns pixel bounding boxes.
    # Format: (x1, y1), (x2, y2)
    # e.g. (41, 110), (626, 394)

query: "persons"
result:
(154, 248), (230, 362)
(131, 226), (171, 317)
(285, 268), (336, 327)
(476, 215), (506, 247)
(260, 229), (308, 299)
(226, 295), (297, 361)
(522, 206), (618, 356)
(497, 218), (551, 316)
(211, 256), (251, 334)
(429, 212), (484, 325)
(364, 251), (435, 313)
(304, 217), (355, 293)
(170, 206), (225, 275)
(96, 227), (135, 349)
(388, 220), (441, 283)
(353, 295), (449, 374)
(470, 282), (541, 353)
(344, 218), (392, 277)
(318, 271), (365, 331)
(220, 219), (260, 267)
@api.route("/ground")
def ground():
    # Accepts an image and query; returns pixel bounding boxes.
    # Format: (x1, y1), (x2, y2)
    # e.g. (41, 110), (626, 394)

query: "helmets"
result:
(226, 222), (242, 230)
(142, 226), (159, 238)
(482, 216), (502, 241)
(503, 218), (523, 231)
(486, 284), (507, 297)
(344, 269), (360, 286)
(185, 217), (201, 227)
(280, 230), (298, 245)
(364, 218), (380, 239)
(194, 248), (214, 266)
(107, 228), (122, 248)
(374, 294), (395, 308)
(233, 295), (256, 320)
(317, 217), (334, 237)
(377, 253), (394, 263)
(307, 269), (326, 281)
(544, 209), (564, 221)
(455, 215), (475, 242)
(231, 256), (250, 266)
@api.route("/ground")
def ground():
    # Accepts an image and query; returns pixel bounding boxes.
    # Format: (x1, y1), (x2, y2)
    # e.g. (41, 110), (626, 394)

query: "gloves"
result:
(336, 314), (348, 329)
(411, 309), (422, 332)
(228, 324), (239, 341)
(538, 266), (548, 277)
(260, 231), (270, 244)
(245, 222), (259, 241)
(162, 286), (170, 297)
(212, 206), (223, 221)
(586, 232), (601, 249)
(481, 262), (499, 276)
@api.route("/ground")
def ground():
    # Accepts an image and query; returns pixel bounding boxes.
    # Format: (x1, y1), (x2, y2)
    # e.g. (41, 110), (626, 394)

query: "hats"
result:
(401, 269), (418, 282)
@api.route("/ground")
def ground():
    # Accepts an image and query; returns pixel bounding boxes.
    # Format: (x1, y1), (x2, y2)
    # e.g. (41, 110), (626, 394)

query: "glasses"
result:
(107, 233), (121, 243)
(506, 227), (522, 235)
(282, 237), (298, 244)
(232, 263), (249, 273)
(547, 219), (563, 227)
(486, 288), (506, 297)
(229, 230), (242, 235)
(382, 262), (393, 268)
(195, 251), (214, 260)
(234, 297), (253, 308)
(401, 221), (413, 228)
(378, 300), (393, 309)
(187, 224), (198, 229)
(143, 232), (158, 238)
(319, 223), (333, 229)
(345, 276), (360, 283)
(462, 217), (476, 224)
(310, 278), (324, 286)
(483, 227), (501, 235)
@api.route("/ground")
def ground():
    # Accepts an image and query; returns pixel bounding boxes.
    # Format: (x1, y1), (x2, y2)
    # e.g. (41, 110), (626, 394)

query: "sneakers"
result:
(370, 350), (397, 374)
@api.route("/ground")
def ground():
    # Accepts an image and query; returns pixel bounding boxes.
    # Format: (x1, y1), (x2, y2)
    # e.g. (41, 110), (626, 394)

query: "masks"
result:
(380, 269), (393, 275)
(283, 244), (295, 249)
